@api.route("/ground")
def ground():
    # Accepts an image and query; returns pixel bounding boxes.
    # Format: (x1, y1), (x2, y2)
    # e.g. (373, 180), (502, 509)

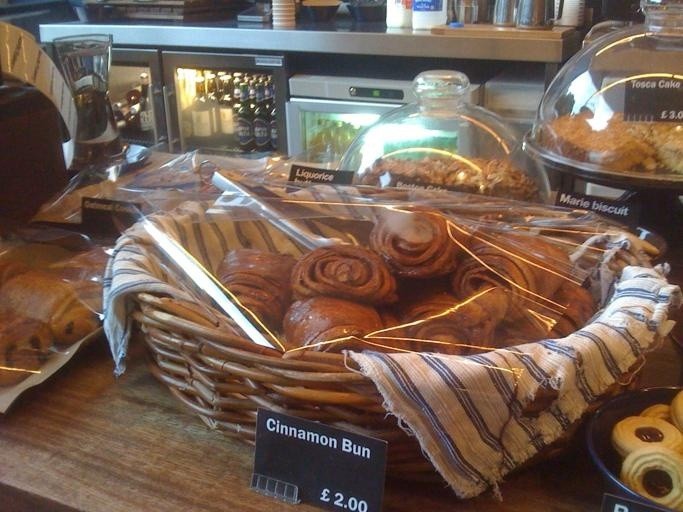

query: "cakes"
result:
(611, 391), (683, 512)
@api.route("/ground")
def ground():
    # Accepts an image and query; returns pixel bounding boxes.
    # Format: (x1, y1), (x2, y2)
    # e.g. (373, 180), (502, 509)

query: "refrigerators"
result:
(286, 74), (477, 170)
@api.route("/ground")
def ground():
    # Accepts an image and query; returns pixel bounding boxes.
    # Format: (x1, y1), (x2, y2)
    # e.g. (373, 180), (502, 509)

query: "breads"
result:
(542, 111), (683, 174)
(0, 243), (107, 386)
(214, 200), (596, 353)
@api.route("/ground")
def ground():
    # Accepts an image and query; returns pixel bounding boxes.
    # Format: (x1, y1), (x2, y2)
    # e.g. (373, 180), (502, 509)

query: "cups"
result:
(271, 1), (296, 27)
(52, 33), (126, 169)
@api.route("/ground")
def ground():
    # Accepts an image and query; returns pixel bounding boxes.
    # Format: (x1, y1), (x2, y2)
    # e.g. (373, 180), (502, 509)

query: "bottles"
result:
(384, 0), (588, 29)
(111, 71), (153, 140)
(311, 120), (368, 171)
(184, 70), (276, 151)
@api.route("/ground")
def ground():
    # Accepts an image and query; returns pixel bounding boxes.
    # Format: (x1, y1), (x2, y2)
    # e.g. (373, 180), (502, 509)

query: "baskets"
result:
(131, 187), (665, 471)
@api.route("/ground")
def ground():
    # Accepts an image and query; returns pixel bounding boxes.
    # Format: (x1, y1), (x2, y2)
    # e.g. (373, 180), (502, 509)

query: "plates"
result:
(586, 383), (679, 512)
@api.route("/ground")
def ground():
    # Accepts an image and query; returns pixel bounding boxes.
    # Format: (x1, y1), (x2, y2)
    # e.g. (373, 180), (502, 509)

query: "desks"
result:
(0, 148), (681, 512)
